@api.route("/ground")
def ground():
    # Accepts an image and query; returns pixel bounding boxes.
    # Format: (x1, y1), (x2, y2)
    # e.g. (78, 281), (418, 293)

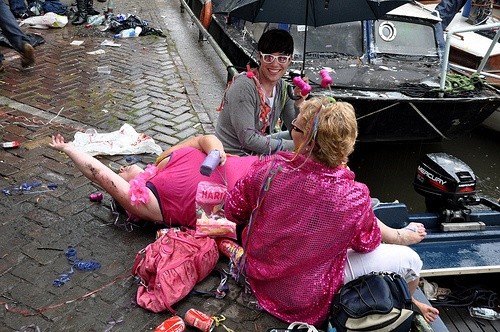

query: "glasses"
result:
(260, 52), (291, 64)
(289, 120), (304, 136)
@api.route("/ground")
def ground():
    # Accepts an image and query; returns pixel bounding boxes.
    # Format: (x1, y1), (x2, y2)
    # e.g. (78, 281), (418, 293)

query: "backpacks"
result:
(331, 271), (424, 332)
(43, 1), (67, 15)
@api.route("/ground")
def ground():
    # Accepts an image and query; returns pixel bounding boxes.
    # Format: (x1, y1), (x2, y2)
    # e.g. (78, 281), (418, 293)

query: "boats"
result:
(417, 0), (500, 68)
(191, 0), (500, 147)
(373, 149), (500, 332)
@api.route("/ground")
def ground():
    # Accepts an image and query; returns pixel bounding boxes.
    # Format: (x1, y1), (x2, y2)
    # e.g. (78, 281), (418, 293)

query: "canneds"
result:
(183, 308), (216, 332)
(154, 316), (186, 332)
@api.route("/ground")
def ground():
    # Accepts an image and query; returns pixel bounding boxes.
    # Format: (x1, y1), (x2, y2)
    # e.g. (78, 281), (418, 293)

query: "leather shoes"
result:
(72, 15), (87, 24)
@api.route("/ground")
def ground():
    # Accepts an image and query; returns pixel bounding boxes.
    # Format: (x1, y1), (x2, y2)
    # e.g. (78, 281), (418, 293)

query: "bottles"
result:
(114, 28), (135, 38)
(470, 306), (500, 321)
(87, 13), (103, 23)
(107, 0), (113, 13)
(85, 15), (106, 28)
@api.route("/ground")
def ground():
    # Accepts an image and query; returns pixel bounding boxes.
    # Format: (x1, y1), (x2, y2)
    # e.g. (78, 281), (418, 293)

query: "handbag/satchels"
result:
(130, 226), (219, 313)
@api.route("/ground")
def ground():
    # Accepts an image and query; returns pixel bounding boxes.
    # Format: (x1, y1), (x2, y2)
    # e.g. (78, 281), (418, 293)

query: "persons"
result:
(47, 134), (427, 247)
(72, 0), (100, 25)
(224, 96), (439, 323)
(215, 28), (380, 208)
(0, 0), (36, 70)
